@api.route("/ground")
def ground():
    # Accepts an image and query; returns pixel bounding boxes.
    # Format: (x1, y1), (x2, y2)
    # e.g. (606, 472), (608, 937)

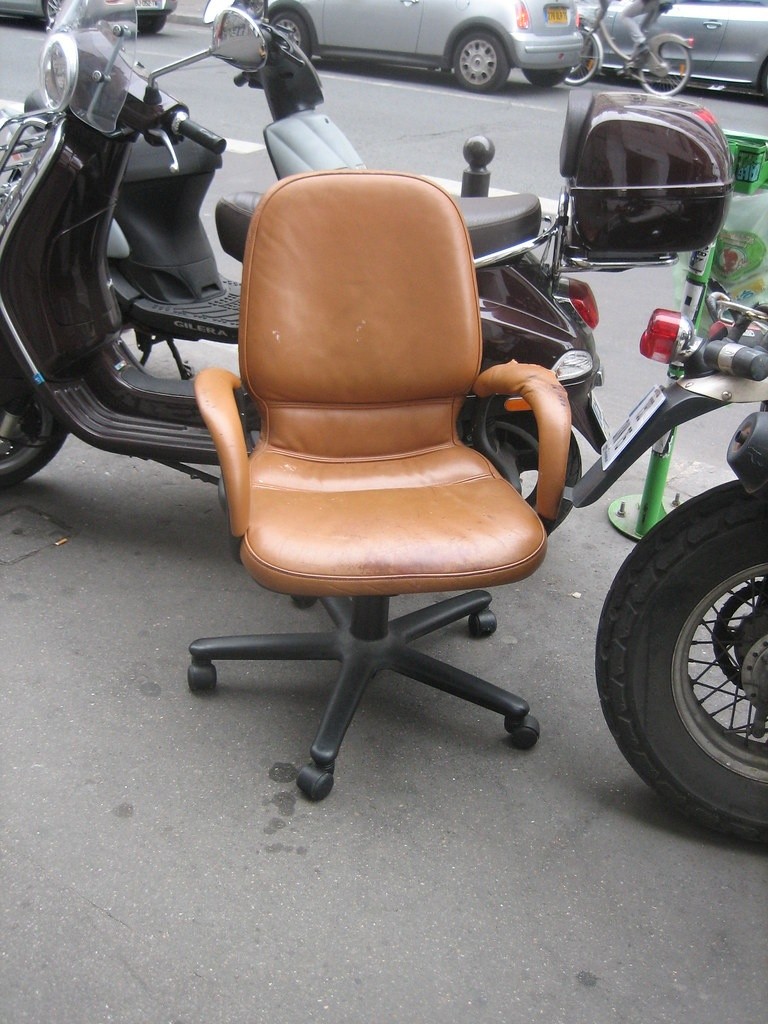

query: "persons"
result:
(620, 1), (674, 64)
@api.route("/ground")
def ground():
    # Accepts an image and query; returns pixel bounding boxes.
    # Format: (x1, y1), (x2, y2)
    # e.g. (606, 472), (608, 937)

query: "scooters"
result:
(1, 2), (734, 491)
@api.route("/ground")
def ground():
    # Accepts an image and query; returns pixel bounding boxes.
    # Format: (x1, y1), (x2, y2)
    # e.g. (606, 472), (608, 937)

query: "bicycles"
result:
(564, 1), (693, 98)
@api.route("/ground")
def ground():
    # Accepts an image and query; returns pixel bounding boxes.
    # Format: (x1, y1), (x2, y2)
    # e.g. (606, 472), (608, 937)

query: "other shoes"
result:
(615, 65), (640, 79)
(631, 43), (649, 62)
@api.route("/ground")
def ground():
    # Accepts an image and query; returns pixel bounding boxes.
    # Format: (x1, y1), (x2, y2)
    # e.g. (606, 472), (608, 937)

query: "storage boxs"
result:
(567, 94), (734, 255)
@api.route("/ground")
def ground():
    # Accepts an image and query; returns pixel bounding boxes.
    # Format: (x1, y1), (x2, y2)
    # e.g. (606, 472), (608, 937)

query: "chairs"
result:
(188, 171), (571, 802)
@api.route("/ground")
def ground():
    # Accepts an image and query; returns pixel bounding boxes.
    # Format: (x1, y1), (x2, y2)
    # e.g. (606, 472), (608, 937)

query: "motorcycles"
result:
(573, 293), (768, 847)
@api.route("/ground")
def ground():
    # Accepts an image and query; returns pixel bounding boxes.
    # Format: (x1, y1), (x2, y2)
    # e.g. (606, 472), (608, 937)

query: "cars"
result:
(0, 1), (768, 104)
